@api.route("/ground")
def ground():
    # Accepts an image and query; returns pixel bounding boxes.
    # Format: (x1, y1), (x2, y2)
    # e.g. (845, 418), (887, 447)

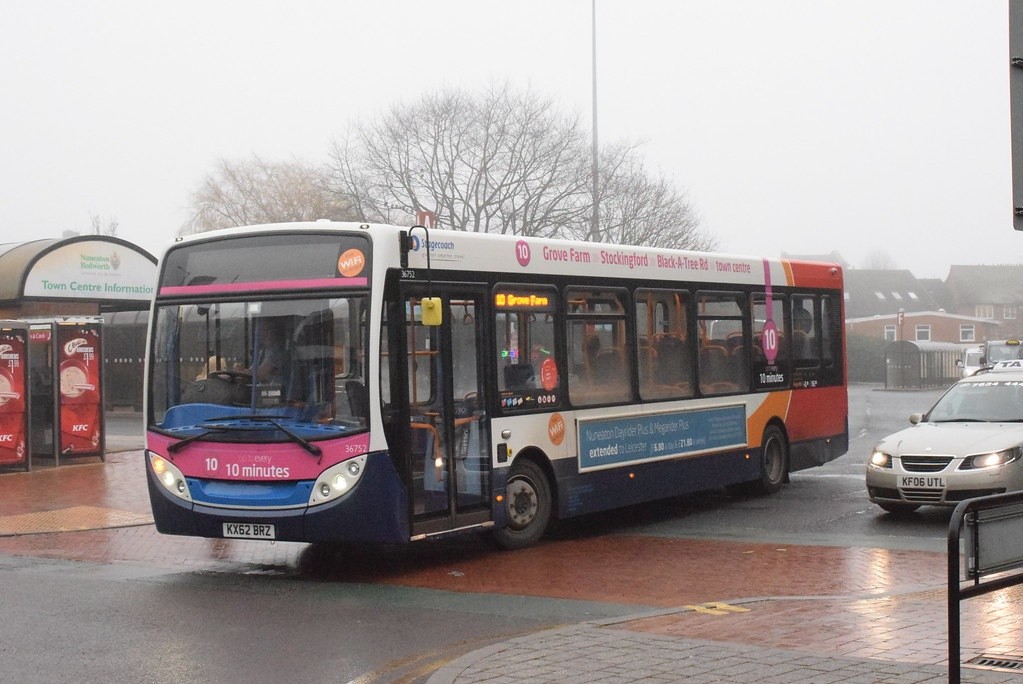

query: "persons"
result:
(230, 317), (304, 410)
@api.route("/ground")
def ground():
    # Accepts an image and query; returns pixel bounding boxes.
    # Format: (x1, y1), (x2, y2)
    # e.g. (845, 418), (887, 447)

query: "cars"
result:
(865, 370), (1023, 516)
(954, 338), (1023, 382)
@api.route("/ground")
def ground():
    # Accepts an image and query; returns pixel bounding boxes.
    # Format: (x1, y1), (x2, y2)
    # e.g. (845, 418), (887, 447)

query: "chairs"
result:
(597, 329), (807, 393)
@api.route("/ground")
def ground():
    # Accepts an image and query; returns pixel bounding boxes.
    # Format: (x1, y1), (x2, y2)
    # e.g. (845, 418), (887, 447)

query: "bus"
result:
(142, 218), (852, 554)
